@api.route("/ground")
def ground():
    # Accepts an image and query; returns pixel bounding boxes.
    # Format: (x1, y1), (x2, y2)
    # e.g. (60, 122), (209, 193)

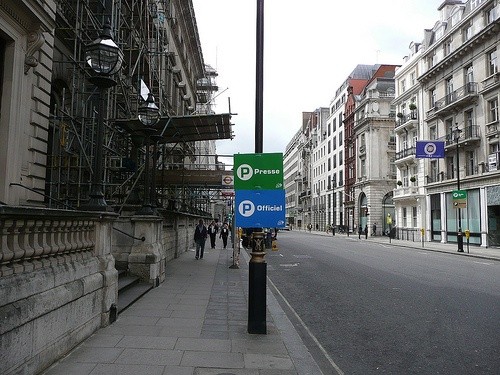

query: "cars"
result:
(281, 223), (290, 231)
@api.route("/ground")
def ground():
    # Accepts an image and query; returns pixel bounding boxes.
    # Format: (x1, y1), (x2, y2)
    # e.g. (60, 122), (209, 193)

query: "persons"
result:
(219, 223), (229, 248)
(208, 221), (218, 249)
(194, 219), (208, 259)
(371, 223), (376, 236)
(357, 224), (368, 239)
(308, 223), (312, 232)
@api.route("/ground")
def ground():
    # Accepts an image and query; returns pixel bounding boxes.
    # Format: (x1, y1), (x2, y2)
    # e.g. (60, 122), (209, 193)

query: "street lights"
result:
(452, 125), (464, 252)
(331, 179), (336, 235)
(84, 18), (125, 210)
(136, 91), (163, 215)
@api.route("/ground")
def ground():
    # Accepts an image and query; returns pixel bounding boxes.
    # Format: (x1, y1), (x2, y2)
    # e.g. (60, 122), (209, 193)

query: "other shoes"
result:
(200, 256), (204, 259)
(195, 255), (199, 260)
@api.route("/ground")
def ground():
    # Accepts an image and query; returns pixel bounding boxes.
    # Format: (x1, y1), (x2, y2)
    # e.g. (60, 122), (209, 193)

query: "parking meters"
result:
(464, 230), (470, 253)
